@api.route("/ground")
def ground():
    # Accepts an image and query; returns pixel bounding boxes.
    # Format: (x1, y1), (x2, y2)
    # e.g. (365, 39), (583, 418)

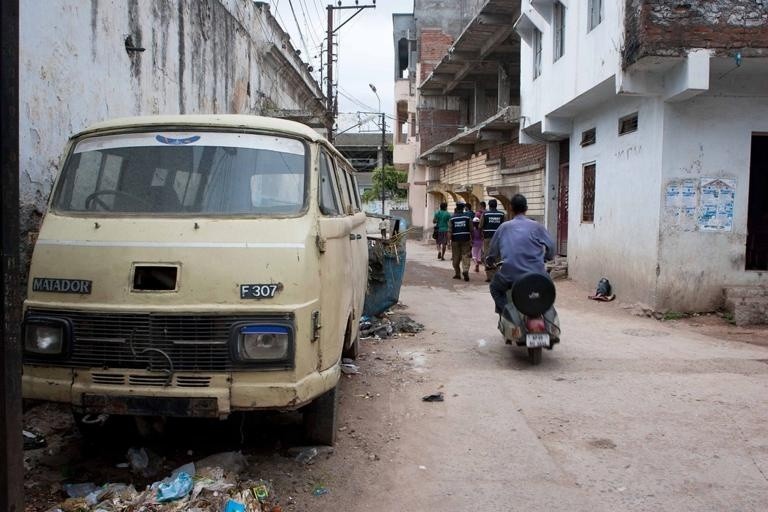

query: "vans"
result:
(21, 113), (369, 447)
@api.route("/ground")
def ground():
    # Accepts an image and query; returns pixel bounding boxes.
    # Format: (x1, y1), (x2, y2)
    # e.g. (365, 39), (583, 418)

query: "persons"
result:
(433, 199), (504, 282)
(378, 217), (387, 238)
(485, 193), (555, 314)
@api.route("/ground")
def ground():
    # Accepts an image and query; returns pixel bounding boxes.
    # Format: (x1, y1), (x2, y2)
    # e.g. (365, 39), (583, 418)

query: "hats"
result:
(472, 216), (480, 222)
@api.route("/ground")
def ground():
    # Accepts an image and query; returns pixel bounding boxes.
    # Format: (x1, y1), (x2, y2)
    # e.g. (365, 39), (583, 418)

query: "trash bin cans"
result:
(364, 211), (405, 318)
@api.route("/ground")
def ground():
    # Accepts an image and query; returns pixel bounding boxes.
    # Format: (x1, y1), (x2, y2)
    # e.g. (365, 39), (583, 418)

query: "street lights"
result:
(368, 82), (381, 129)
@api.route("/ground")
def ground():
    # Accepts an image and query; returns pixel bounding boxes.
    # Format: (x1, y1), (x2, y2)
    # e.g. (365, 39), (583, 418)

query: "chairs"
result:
(145, 186), (180, 214)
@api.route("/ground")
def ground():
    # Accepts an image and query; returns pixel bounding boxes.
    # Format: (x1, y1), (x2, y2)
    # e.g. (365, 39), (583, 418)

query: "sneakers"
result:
(476, 261), (482, 272)
(453, 271), (469, 281)
(438, 252), (445, 260)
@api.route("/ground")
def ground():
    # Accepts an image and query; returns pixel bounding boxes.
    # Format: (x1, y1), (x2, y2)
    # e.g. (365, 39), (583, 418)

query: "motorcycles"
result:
(484, 255), (559, 366)
